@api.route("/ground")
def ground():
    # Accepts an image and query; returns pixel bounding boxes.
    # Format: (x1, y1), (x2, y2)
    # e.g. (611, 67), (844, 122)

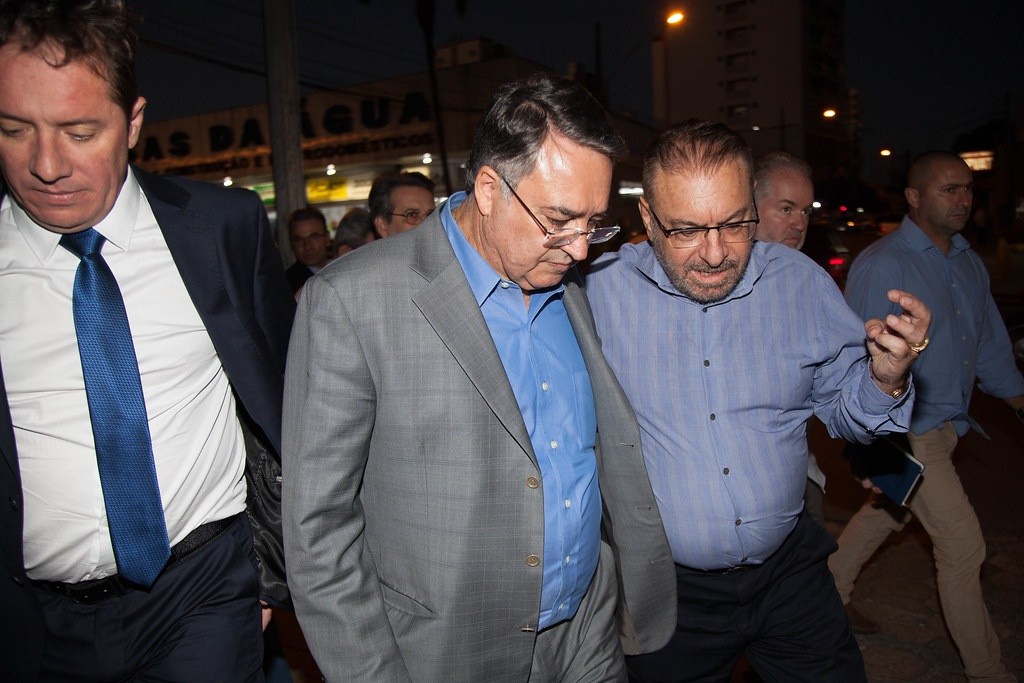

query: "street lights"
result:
(592, 8), (689, 102)
(776, 103), (839, 154)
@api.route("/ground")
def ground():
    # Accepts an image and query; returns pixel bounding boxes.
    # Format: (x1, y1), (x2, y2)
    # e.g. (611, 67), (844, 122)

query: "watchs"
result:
(890, 379), (910, 400)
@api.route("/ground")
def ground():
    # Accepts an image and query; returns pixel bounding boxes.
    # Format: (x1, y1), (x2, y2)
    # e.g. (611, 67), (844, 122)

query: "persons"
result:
(284, 208), (377, 305)
(750, 151), (890, 494)
(0, 0), (299, 683)
(282, 76), (677, 682)
(827, 154), (1024, 683)
(369, 172), (437, 238)
(584, 120), (930, 683)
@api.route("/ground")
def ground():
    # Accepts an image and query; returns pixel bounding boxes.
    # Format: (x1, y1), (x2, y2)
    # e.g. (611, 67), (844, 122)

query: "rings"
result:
(907, 335), (930, 352)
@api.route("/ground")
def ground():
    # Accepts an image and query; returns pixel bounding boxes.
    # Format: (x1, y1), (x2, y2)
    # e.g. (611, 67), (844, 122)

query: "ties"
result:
(59, 228), (173, 590)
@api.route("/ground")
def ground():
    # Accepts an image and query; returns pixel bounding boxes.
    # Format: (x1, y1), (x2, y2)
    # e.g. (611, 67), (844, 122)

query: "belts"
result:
(30, 513), (241, 606)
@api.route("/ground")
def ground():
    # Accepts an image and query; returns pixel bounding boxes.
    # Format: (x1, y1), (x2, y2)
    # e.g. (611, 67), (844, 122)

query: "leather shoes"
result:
(845, 602), (880, 634)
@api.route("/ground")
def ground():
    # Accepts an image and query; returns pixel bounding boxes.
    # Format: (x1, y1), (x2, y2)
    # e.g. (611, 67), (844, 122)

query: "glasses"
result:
(292, 233), (325, 246)
(484, 163), (622, 247)
(389, 212), (428, 225)
(649, 194), (760, 248)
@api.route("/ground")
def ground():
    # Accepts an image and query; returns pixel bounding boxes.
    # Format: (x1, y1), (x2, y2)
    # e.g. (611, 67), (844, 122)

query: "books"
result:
(842, 436), (925, 505)
